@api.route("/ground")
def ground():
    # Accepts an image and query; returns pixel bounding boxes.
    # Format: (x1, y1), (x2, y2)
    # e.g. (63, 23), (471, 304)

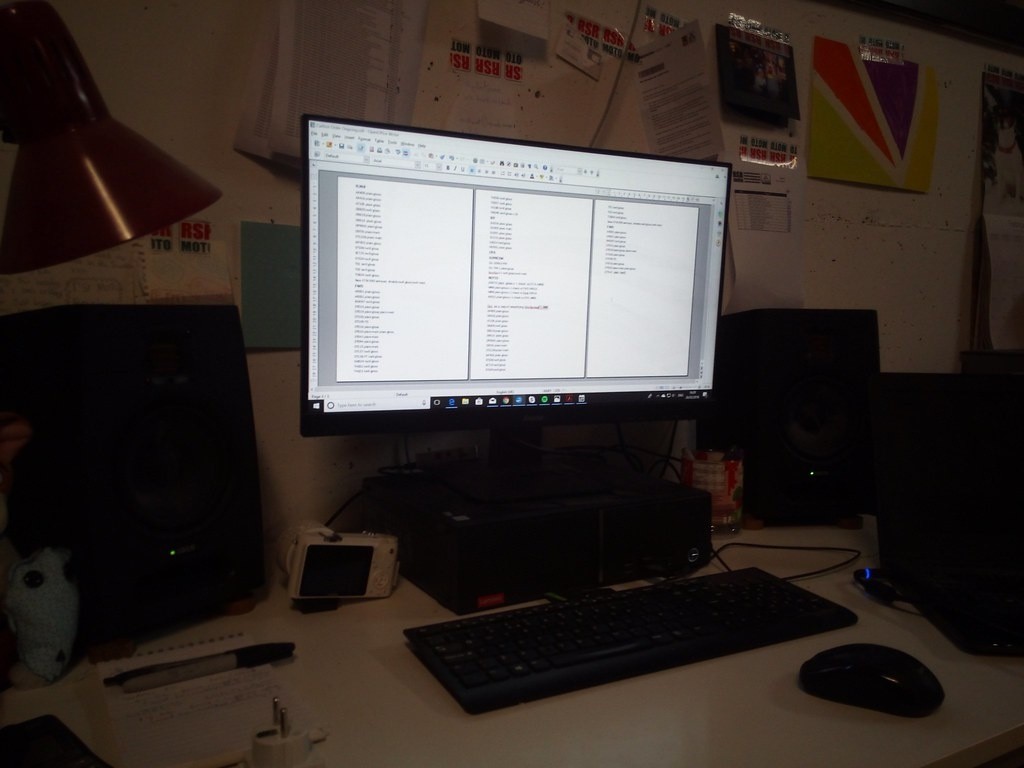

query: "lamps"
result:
(0, 0), (224, 279)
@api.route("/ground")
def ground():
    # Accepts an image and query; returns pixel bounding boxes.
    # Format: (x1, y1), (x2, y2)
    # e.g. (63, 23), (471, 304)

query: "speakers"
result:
(0, 302), (268, 651)
(694, 306), (882, 526)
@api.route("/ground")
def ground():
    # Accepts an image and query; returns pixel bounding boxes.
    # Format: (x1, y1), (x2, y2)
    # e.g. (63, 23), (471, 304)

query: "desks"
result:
(0, 515), (1024, 768)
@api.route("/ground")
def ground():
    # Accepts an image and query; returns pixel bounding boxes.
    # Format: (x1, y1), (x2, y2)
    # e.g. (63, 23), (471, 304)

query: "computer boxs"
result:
(360, 448), (713, 616)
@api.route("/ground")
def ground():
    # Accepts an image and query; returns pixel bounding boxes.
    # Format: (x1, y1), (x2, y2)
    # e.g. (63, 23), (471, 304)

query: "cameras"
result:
(290, 531), (398, 600)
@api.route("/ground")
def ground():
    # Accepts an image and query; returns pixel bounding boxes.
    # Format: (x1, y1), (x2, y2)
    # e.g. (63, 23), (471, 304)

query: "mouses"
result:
(800, 644), (946, 719)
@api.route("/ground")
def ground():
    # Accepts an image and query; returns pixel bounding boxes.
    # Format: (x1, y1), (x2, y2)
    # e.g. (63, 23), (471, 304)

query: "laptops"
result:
(871, 371), (1024, 656)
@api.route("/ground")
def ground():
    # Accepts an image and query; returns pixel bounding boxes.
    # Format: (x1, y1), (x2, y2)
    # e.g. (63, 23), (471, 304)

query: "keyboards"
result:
(404, 567), (858, 715)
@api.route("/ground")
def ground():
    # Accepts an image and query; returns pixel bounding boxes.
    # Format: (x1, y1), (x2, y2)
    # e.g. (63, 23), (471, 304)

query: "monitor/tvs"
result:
(299, 112), (733, 498)
